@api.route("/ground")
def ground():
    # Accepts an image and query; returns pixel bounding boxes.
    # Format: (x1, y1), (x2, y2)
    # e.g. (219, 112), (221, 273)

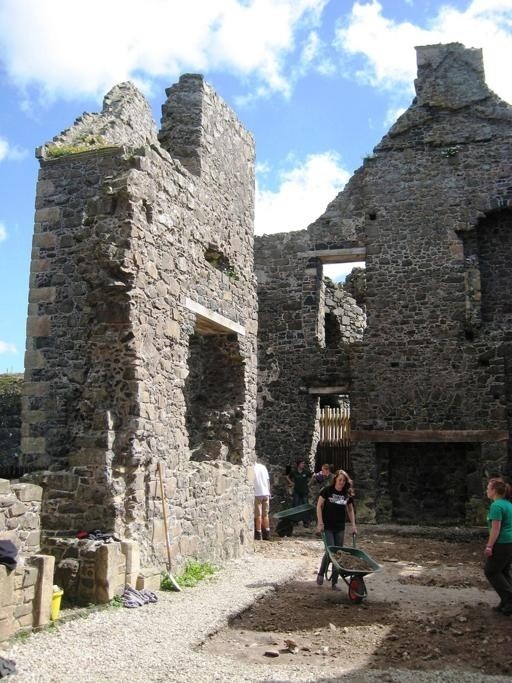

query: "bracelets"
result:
(485, 544), (492, 548)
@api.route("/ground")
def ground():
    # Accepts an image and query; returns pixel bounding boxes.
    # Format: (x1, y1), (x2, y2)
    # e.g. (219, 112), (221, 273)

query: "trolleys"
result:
(271, 490), (322, 537)
(320, 528), (380, 605)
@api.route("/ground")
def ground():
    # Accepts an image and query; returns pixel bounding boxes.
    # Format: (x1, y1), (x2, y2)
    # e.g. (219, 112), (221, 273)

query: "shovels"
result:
(157, 461), (184, 591)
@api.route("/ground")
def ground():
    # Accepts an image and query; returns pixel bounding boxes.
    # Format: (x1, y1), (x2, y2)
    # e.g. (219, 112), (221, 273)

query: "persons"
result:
(482, 478), (512, 616)
(254, 452), (273, 541)
(285, 458), (316, 527)
(310, 463), (339, 537)
(316, 469), (359, 592)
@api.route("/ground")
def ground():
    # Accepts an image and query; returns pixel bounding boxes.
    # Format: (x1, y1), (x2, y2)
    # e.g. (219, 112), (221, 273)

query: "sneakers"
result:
(317, 575), (324, 585)
(493, 601), (512, 616)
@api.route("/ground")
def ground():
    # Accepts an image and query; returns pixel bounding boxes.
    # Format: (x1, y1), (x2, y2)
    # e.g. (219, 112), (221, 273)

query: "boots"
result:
(255, 529), (261, 540)
(262, 528), (269, 540)
